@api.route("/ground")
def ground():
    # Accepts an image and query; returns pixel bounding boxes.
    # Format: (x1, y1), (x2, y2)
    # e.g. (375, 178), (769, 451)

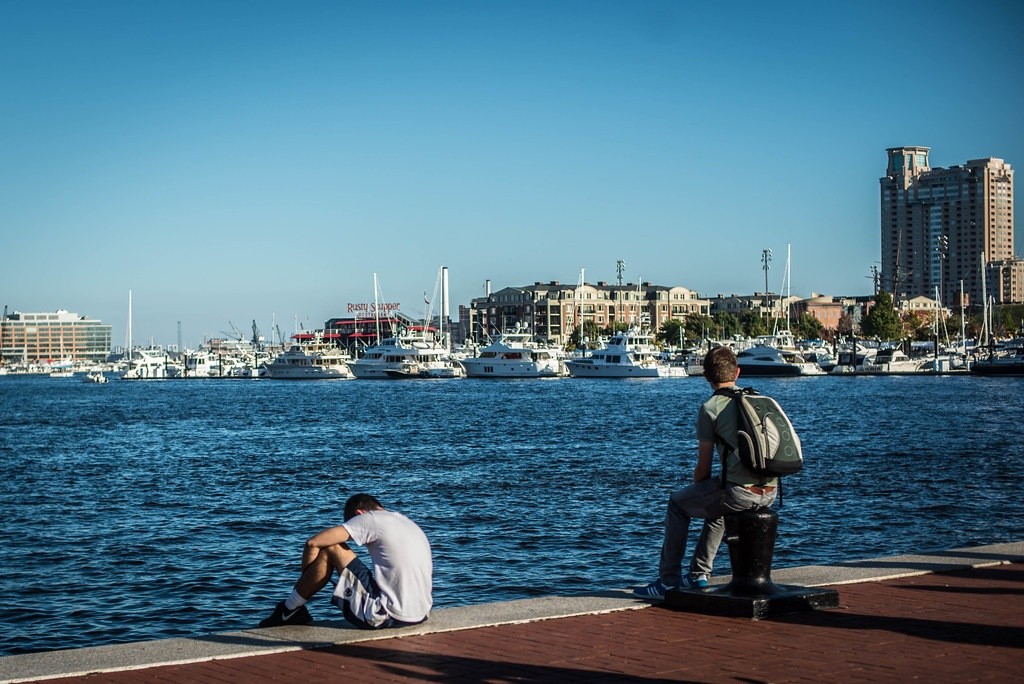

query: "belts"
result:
(729, 481), (775, 495)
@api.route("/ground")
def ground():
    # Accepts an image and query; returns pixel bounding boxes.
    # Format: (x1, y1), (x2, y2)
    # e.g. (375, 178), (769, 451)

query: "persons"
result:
(258, 493), (435, 630)
(631, 345), (779, 599)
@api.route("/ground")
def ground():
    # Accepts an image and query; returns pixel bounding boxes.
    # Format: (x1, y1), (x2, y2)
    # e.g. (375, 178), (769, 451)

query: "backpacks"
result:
(712, 387), (803, 477)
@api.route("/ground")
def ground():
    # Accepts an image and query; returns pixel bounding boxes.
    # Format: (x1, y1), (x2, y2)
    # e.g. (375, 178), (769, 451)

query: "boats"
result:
(49, 368), (73, 378)
(86, 368), (110, 383)
(112, 242), (1024, 378)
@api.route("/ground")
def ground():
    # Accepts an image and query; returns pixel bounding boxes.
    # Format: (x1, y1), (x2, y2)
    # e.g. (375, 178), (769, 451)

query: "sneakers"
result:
(258, 599), (313, 628)
(633, 579), (679, 600)
(679, 572), (709, 590)
